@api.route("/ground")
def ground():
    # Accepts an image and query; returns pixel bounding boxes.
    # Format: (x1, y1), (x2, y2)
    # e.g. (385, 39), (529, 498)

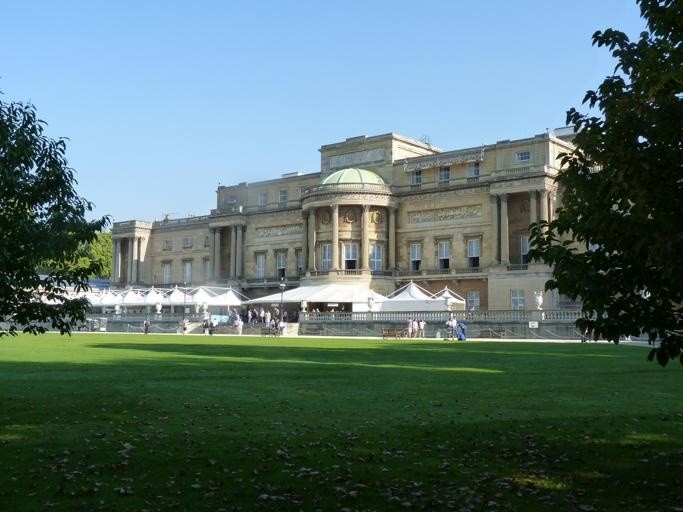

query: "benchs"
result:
(478, 329), (505, 338)
(381, 327), (411, 338)
(260, 325), (284, 337)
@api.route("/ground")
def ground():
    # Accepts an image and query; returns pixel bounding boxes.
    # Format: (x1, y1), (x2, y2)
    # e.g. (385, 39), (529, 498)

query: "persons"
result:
(144, 318), (151, 334)
(302, 307), (320, 320)
(446, 316), (457, 338)
(203, 320), (218, 335)
(183, 318), (189, 334)
(230, 310), (243, 334)
(248, 307), (280, 328)
(407, 317), (426, 338)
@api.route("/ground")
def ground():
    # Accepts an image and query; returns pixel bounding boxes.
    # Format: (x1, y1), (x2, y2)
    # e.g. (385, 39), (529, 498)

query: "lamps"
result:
(477, 147), (486, 161)
